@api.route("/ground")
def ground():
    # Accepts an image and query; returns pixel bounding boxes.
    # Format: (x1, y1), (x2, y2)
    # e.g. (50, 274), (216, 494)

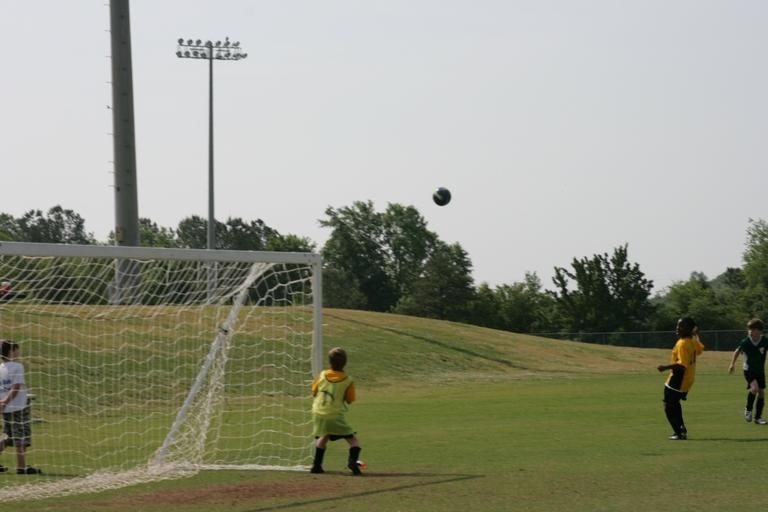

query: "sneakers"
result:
(348, 462), (361, 475)
(754, 418), (767, 424)
(668, 427), (687, 439)
(311, 464), (324, 473)
(744, 405), (752, 422)
(17, 465), (40, 474)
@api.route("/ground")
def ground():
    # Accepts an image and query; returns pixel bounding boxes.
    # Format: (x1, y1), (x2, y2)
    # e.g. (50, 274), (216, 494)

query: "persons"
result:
(658, 316), (704, 439)
(311, 347), (363, 474)
(728, 318), (768, 424)
(1, 340), (42, 475)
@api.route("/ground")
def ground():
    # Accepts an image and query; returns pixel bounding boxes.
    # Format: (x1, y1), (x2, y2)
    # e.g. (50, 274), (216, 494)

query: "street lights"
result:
(177, 33), (249, 302)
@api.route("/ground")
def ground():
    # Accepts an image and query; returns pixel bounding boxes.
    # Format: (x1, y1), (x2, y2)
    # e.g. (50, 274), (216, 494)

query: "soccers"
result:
(433, 187), (450, 205)
